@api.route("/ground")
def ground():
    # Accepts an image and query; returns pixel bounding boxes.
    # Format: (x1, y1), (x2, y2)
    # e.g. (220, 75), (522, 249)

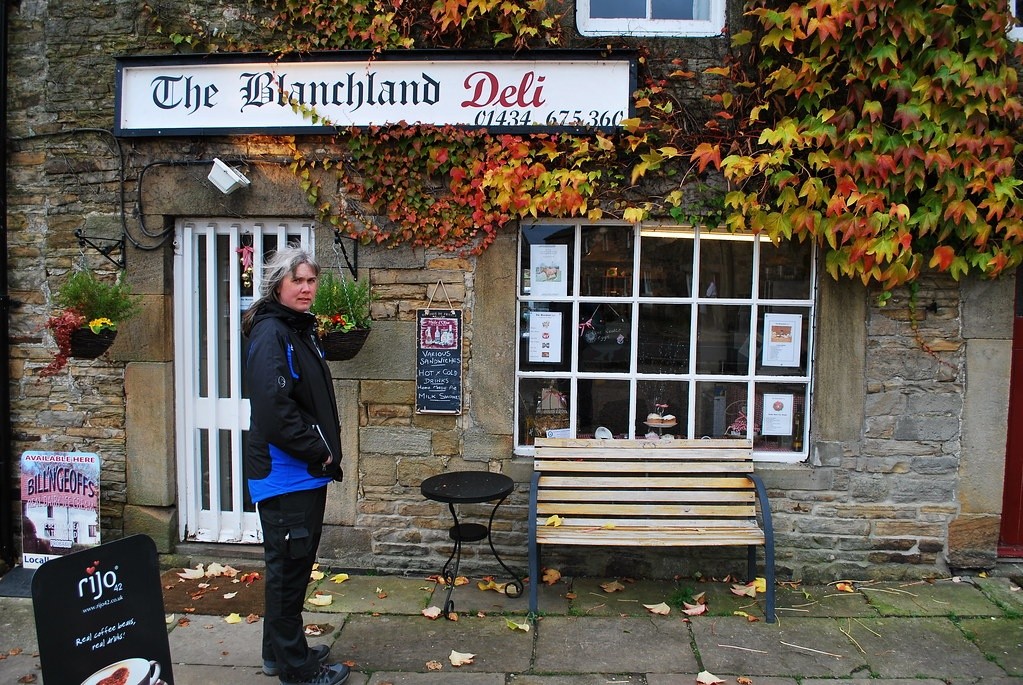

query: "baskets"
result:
(315, 320), (371, 361)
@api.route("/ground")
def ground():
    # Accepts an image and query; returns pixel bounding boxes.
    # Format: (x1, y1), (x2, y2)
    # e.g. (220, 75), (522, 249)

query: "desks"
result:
(728, 384), (804, 442)
(420, 471), (525, 621)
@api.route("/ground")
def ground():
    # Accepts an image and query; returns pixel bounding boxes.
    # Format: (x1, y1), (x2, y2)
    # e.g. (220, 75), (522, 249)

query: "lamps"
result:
(208, 158), (251, 195)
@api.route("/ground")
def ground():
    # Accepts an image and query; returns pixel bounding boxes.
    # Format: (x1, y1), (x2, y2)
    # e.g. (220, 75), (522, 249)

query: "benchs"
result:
(528, 438), (775, 624)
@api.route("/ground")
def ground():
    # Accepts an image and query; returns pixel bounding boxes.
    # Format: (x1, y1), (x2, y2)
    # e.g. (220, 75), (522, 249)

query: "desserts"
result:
(646, 412), (677, 424)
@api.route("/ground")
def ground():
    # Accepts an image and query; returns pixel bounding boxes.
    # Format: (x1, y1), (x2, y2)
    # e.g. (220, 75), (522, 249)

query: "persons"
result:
(240, 250), (350, 685)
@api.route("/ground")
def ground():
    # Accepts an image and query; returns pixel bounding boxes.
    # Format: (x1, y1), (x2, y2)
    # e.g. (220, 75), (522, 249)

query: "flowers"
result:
(310, 269), (380, 338)
(35, 262), (144, 379)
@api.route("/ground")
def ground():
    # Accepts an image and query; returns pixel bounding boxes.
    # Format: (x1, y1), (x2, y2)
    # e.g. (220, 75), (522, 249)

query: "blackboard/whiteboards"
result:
(414, 307), (465, 417)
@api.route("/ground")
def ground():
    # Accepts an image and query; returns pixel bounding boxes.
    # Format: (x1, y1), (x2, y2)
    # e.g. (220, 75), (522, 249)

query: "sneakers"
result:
(262, 644), (329, 675)
(280, 661), (351, 685)
